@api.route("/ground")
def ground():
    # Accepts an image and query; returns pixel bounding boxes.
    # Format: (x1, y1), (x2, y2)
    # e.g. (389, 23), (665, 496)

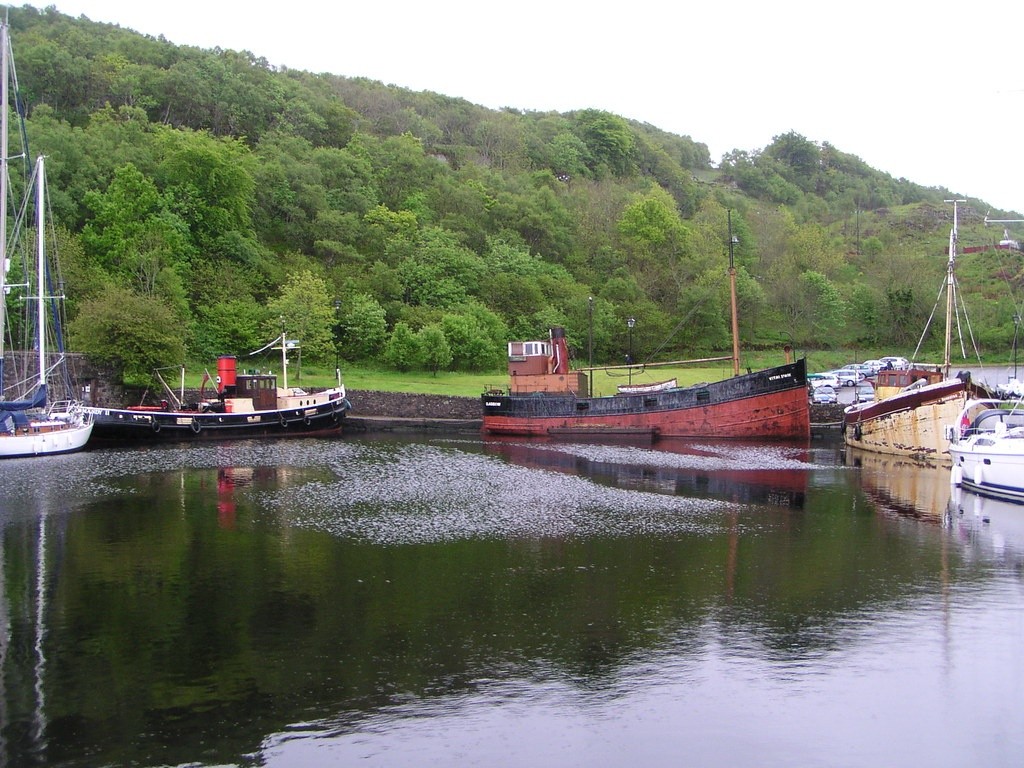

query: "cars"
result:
(840, 363), (875, 381)
(830, 369), (860, 387)
(812, 387), (839, 404)
(863, 360), (887, 375)
(807, 372), (840, 390)
(879, 357), (909, 370)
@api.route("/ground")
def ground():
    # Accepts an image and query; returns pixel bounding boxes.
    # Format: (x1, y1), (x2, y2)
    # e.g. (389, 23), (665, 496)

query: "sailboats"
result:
(843, 199), (1000, 458)
(947, 207), (1024, 505)
(0, 6), (95, 459)
(481, 434), (809, 602)
(481, 207), (812, 442)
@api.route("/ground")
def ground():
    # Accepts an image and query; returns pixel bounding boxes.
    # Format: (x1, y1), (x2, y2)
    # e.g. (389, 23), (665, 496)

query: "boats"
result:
(617, 377), (677, 395)
(77, 315), (351, 439)
(1000, 229), (1020, 250)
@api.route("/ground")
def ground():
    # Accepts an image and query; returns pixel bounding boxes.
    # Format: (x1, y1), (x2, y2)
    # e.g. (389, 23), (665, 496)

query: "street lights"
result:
(335, 298), (341, 378)
(1012, 311), (1021, 379)
(627, 317), (635, 385)
(588, 297), (594, 397)
